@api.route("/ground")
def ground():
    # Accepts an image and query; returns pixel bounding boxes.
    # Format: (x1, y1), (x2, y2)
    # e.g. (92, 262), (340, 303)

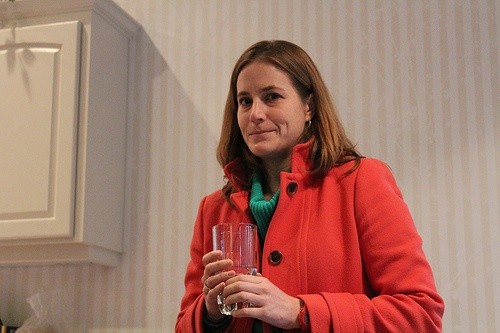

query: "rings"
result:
(202, 280), (212, 293)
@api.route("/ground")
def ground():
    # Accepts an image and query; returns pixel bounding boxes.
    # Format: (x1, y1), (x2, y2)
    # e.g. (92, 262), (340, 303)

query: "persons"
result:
(175, 39), (445, 332)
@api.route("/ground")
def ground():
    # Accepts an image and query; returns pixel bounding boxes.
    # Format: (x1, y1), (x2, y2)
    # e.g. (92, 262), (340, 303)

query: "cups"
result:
(212, 222), (259, 316)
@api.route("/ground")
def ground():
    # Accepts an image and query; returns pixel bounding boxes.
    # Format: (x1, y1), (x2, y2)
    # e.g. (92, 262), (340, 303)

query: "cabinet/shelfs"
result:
(1, 0), (140, 267)
